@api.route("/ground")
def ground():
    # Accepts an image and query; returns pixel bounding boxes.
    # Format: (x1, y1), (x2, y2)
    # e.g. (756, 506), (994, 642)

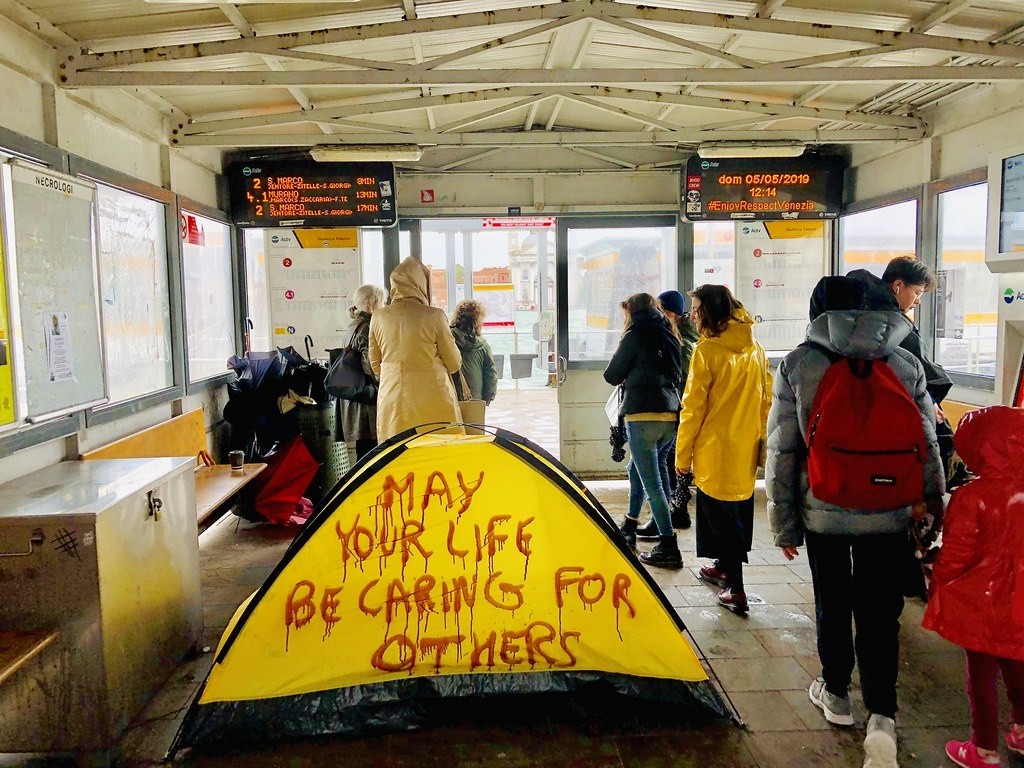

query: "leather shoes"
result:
(717, 587), (749, 612)
(699, 562), (730, 588)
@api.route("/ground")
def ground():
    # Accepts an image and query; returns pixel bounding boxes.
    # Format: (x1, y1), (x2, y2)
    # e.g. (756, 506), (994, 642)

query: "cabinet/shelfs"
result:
(0, 456), (204, 744)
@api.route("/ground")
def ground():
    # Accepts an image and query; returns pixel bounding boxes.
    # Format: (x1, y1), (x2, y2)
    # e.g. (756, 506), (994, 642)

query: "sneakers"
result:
(946, 739), (1001, 768)
(809, 677), (853, 725)
(863, 714), (898, 768)
(1006, 725), (1024, 753)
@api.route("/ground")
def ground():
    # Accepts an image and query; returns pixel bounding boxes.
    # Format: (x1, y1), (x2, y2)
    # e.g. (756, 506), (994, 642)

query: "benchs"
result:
(77, 407), (268, 544)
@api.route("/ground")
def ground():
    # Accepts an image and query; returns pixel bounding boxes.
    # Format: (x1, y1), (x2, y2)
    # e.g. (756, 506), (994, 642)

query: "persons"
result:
(764, 275), (945, 768)
(368, 257), (466, 445)
(921, 404), (1024, 768)
(675, 284), (774, 613)
(845, 256), (953, 424)
(334, 285), (387, 461)
(601, 291), (700, 569)
(450, 300), (497, 407)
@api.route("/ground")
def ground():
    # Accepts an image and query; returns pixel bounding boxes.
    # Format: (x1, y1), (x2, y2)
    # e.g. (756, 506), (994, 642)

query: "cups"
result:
(228, 450), (244, 470)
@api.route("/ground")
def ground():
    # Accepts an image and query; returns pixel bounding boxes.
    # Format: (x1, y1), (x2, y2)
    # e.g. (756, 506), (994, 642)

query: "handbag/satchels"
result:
(324, 346), (379, 404)
(458, 399), (485, 435)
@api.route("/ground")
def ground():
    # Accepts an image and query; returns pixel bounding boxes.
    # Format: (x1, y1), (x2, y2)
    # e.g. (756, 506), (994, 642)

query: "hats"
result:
(658, 291), (684, 316)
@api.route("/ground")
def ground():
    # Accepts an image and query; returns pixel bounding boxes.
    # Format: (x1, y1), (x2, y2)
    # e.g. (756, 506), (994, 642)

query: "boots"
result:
(638, 532), (683, 570)
(619, 514), (637, 547)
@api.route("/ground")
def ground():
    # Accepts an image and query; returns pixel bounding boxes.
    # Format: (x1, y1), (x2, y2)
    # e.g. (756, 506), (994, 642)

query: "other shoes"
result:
(638, 517), (660, 538)
(671, 513), (692, 529)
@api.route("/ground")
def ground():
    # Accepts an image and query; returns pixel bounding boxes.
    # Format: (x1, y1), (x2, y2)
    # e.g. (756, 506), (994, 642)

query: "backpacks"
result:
(797, 341), (926, 508)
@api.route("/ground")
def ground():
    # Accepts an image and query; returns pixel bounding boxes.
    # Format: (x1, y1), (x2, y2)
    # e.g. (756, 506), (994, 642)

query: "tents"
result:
(164, 421), (736, 763)
